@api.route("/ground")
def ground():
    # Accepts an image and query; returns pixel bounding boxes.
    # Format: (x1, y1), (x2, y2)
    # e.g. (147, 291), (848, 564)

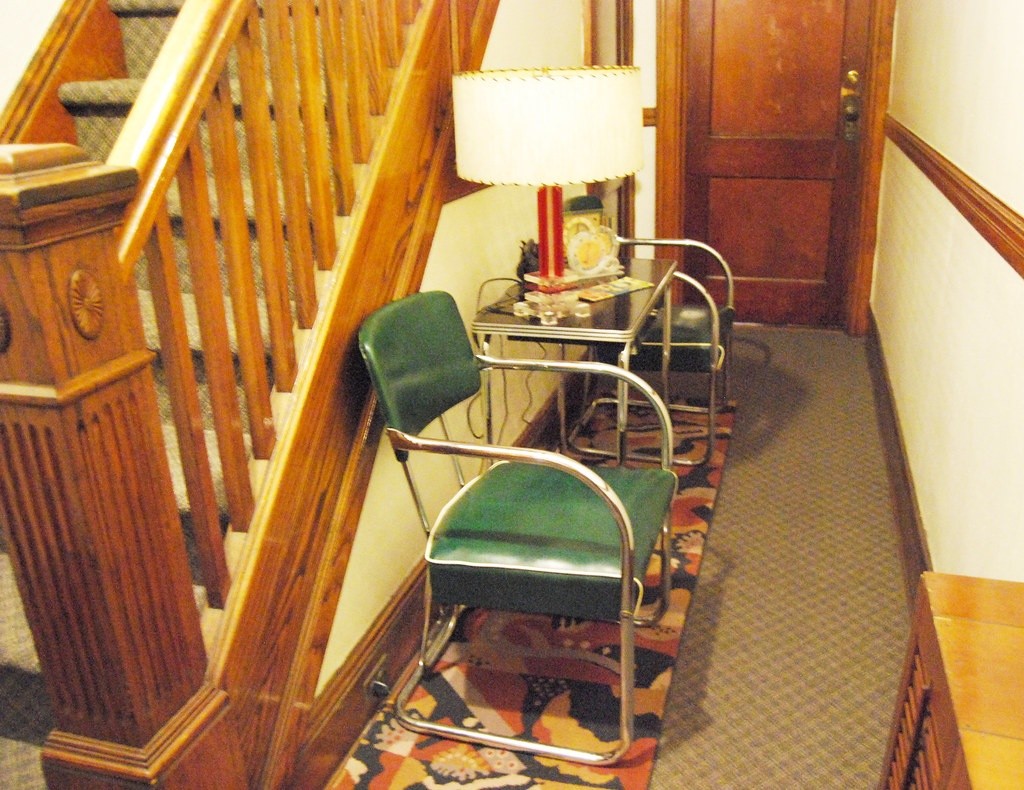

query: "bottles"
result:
(538, 274), (560, 326)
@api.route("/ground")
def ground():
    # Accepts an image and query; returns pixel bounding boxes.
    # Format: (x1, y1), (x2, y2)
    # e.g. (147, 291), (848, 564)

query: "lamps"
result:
(451, 65), (647, 326)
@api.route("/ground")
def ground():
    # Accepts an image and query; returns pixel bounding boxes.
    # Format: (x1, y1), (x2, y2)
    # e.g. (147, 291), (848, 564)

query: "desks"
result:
(471, 258), (680, 469)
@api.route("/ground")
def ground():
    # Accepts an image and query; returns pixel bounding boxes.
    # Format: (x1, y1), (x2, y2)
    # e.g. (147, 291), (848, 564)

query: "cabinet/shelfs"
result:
(878, 569), (1024, 790)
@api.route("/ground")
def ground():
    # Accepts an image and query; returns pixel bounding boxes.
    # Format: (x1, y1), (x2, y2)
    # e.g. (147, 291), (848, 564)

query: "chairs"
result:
(568, 237), (737, 466)
(356, 290), (682, 768)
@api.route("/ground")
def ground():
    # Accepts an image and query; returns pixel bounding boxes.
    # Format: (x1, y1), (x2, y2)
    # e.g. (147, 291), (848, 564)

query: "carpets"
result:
(319, 397), (741, 790)
